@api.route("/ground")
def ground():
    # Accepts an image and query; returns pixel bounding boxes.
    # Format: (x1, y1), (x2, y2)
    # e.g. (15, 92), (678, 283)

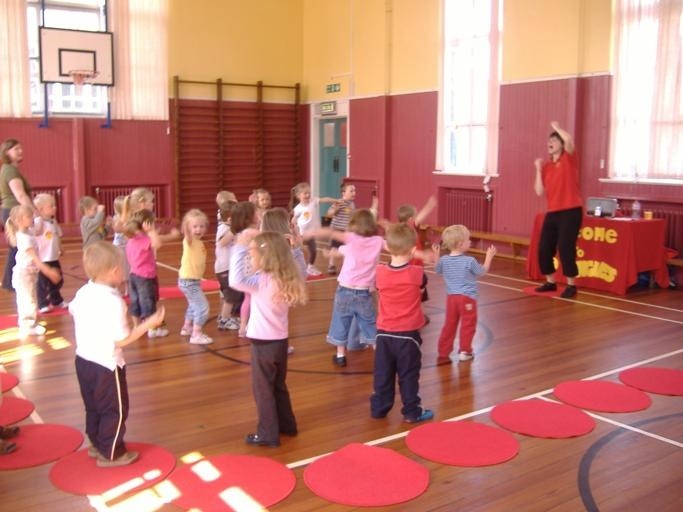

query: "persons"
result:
(0, 138), (36, 289)
(77, 186), (497, 370)
(228, 227), (307, 445)
(534, 120), (583, 296)
(5, 193), (69, 335)
(69, 241), (165, 465)
(367, 221), (443, 416)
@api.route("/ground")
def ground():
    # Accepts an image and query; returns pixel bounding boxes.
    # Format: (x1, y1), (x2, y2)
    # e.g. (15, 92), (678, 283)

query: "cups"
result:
(643, 211), (652, 220)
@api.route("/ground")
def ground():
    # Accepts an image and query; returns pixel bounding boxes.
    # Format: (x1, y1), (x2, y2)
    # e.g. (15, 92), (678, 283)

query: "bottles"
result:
(594, 202), (601, 218)
(631, 198), (640, 219)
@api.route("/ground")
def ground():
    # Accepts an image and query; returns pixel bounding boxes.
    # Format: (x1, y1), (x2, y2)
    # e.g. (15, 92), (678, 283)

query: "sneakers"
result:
(180, 325), (215, 346)
(244, 431), (280, 445)
(147, 328), (170, 339)
(216, 313), (241, 332)
(0, 438), (17, 456)
(559, 284), (576, 297)
(331, 353), (347, 367)
(534, 280), (557, 291)
(306, 264), (336, 275)
(0, 424), (20, 438)
(457, 349), (473, 361)
(402, 408), (433, 423)
(87, 442), (139, 467)
(18, 299), (70, 335)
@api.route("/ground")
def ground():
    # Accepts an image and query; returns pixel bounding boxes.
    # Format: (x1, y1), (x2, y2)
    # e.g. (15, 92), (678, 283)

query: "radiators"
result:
(442, 191), (485, 235)
(28, 188), (61, 218)
(618, 206), (682, 259)
(98, 186), (160, 222)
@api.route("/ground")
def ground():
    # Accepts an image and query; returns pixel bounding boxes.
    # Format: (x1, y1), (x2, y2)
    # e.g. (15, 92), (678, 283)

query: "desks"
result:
(525, 209), (672, 297)
